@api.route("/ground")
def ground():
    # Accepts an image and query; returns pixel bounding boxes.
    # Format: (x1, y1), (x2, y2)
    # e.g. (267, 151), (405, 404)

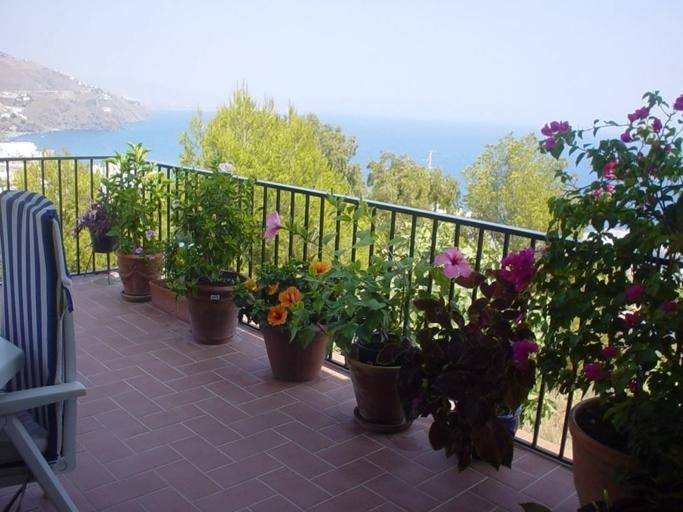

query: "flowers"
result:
(263, 181), (474, 362)
(500, 91), (683, 436)
(234, 256), (347, 343)
(416, 244), (541, 471)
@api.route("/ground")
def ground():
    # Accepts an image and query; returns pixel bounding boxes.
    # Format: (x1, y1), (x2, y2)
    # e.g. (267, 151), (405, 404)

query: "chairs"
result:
(0, 188), (86, 512)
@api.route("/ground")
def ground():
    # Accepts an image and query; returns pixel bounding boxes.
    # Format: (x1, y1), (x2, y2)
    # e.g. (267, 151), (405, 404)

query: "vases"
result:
(346, 334), (431, 434)
(263, 323), (329, 383)
(465, 408), (518, 463)
(566, 393), (683, 511)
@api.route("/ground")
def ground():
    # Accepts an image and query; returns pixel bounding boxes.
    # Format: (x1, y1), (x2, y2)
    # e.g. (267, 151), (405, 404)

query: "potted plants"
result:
(164, 159), (258, 344)
(99, 141), (174, 303)
(71, 199), (127, 283)
(147, 209), (201, 323)
